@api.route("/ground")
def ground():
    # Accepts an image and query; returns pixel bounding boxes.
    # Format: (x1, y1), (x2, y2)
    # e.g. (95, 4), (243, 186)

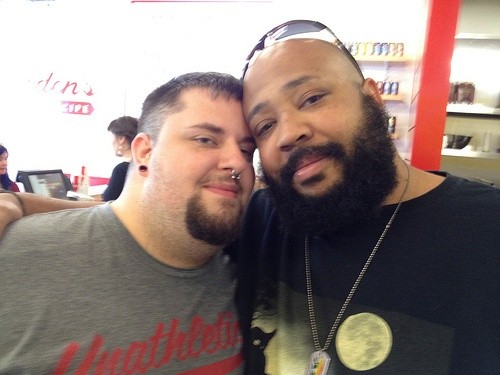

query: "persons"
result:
(0, 18), (500, 375)
(0, 72), (258, 375)
(85, 116), (138, 202)
(0, 145), (20, 192)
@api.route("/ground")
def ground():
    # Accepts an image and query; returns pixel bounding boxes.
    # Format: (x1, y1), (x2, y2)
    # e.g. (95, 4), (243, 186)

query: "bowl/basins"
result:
(443, 134), (473, 149)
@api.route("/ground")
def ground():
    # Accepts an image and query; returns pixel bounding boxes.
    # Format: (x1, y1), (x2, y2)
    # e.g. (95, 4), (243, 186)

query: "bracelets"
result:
(0, 188), (26, 216)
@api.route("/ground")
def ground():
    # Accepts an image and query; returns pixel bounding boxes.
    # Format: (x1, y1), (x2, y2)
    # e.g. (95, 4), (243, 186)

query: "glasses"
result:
(242, 19), (366, 81)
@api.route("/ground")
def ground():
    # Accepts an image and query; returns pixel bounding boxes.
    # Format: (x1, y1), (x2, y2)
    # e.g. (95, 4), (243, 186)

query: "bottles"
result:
(78, 166), (88, 195)
(482, 130), (492, 152)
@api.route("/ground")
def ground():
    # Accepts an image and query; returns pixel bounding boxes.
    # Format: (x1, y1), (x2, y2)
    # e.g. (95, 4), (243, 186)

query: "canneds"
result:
(341, 42), (404, 57)
(375, 81), (398, 95)
(388, 117), (396, 134)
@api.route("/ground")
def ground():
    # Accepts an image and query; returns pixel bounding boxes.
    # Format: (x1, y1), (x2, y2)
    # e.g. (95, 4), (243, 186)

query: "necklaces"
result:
(304, 158), (411, 375)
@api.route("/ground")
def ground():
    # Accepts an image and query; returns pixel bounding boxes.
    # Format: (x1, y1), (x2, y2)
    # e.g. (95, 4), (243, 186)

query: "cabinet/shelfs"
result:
(352, 54), (410, 139)
(443, 31), (500, 159)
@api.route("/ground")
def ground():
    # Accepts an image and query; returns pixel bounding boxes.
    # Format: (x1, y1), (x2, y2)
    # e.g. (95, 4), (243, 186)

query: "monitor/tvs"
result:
(23, 169), (71, 200)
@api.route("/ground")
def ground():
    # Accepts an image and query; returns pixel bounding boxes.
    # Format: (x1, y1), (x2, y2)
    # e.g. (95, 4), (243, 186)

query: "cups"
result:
(71, 175), (79, 192)
